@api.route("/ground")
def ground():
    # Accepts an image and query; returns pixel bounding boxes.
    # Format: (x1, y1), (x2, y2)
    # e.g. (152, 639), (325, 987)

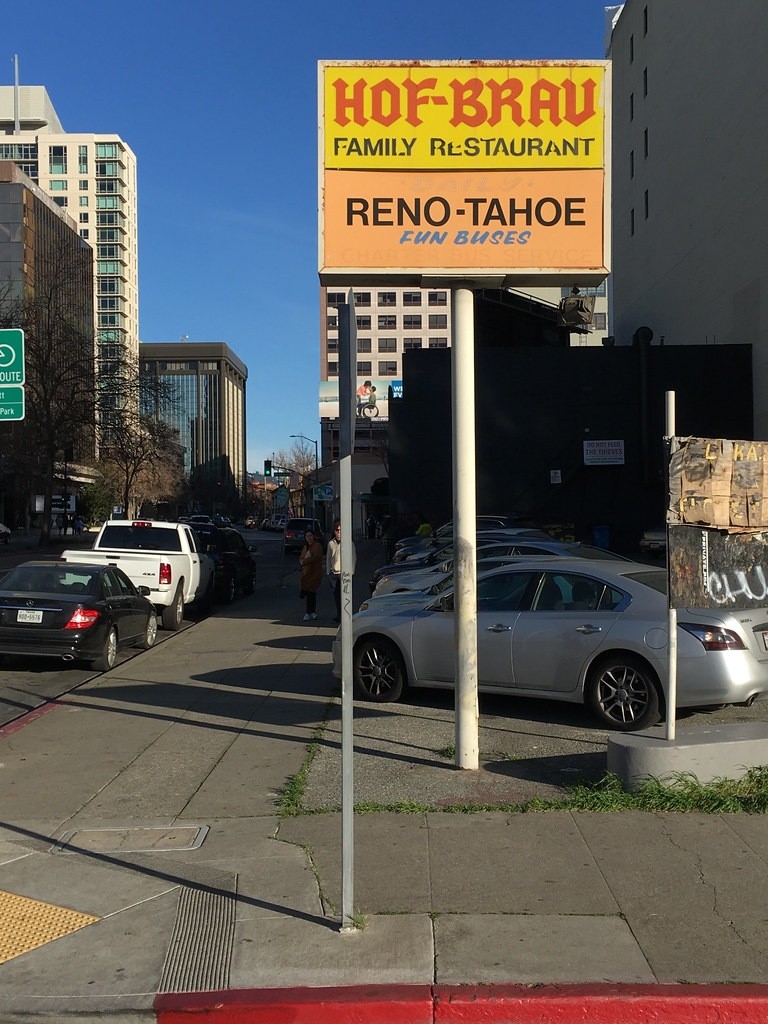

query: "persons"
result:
(299, 531), (325, 621)
(55, 511), (84, 537)
(327, 523), (357, 624)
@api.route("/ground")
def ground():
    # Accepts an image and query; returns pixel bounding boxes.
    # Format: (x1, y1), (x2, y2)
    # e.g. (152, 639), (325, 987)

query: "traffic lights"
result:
(264, 459), (271, 476)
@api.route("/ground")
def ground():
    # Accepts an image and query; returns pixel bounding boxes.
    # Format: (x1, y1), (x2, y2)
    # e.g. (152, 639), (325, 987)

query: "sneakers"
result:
(311, 612), (317, 619)
(302, 612), (310, 620)
(330, 618), (341, 624)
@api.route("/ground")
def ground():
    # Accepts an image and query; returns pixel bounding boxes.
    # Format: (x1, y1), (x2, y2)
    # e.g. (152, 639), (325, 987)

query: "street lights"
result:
(290, 435), (320, 485)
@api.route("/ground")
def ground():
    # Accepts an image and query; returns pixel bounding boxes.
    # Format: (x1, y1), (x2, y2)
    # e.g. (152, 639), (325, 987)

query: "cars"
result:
(342, 506), (766, 736)
(172, 512), (327, 607)
(0, 561), (157, 672)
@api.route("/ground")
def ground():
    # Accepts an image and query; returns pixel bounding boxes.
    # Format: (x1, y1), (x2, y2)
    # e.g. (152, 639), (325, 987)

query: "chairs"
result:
(568, 581), (598, 610)
(516, 578), (563, 613)
(14, 574), (112, 597)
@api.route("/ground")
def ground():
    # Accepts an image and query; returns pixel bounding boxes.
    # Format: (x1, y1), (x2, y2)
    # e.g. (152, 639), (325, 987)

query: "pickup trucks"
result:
(58, 518), (215, 632)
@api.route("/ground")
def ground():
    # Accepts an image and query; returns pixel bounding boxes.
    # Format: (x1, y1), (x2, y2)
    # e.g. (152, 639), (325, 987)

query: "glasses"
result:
(334, 528), (341, 532)
